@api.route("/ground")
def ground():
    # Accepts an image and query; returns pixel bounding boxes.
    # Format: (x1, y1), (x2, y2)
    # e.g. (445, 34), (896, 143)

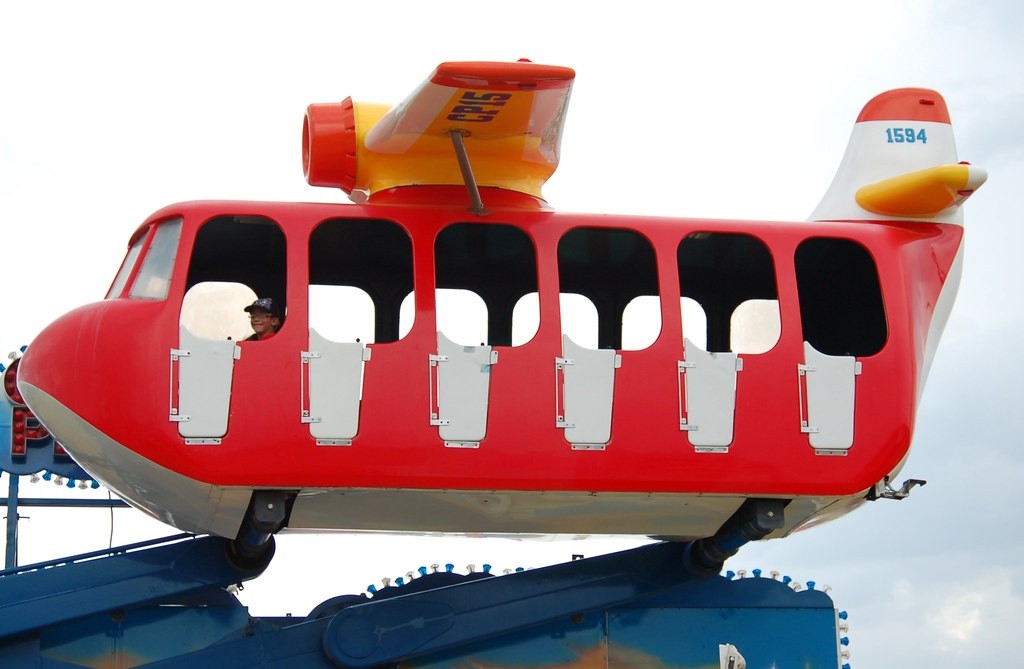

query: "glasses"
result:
(248, 315), (274, 318)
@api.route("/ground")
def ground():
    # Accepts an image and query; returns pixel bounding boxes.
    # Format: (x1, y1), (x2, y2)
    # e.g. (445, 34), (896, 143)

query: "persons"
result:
(241, 297), (281, 341)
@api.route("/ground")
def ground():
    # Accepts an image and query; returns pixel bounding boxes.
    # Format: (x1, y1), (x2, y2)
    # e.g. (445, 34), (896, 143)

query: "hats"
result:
(244, 298), (278, 316)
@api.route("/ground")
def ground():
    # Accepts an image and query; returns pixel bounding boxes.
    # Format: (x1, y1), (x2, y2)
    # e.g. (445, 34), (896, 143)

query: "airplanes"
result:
(13, 55), (988, 565)
